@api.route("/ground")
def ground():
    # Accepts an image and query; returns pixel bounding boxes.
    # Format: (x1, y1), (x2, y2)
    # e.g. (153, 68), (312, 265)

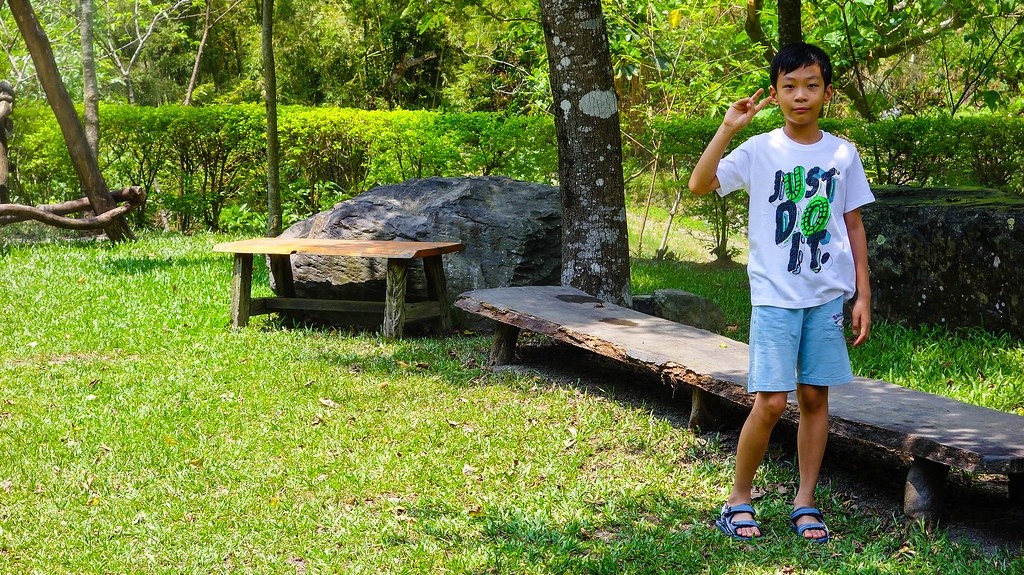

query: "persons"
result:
(688, 42), (876, 543)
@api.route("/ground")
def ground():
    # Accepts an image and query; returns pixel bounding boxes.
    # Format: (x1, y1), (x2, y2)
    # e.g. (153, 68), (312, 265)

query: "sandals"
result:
(715, 501), (764, 541)
(790, 509), (829, 543)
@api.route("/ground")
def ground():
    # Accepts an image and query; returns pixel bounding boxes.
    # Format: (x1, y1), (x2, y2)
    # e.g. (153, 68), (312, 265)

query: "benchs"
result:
(453, 286), (1024, 535)
(212, 237), (464, 340)
